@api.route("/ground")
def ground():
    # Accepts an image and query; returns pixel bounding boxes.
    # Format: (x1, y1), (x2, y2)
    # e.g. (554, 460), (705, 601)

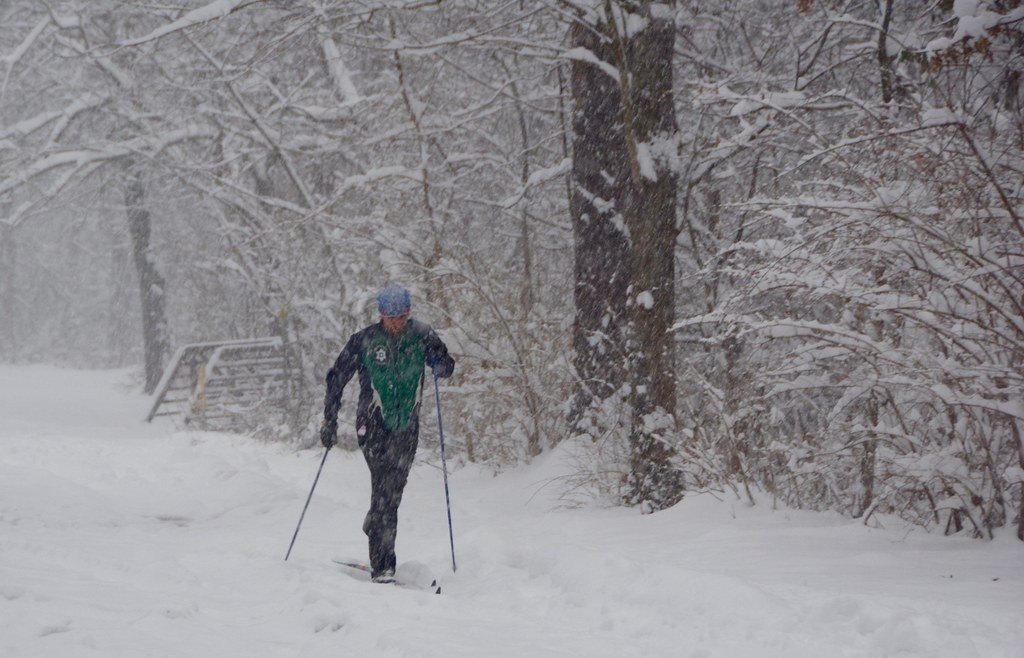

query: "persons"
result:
(319, 284), (455, 588)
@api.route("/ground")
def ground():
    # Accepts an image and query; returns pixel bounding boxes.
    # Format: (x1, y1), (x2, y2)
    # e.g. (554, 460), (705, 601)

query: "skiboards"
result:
(331, 558), (443, 596)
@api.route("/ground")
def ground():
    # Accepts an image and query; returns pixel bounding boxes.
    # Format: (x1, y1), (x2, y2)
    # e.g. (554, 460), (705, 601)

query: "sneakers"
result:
(370, 570), (397, 583)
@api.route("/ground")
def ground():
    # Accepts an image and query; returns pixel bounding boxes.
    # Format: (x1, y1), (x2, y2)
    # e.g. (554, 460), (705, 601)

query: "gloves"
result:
(432, 360), (447, 379)
(320, 421), (338, 447)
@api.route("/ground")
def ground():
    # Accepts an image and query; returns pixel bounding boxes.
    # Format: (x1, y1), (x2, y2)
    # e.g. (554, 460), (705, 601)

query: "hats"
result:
(376, 285), (412, 318)
